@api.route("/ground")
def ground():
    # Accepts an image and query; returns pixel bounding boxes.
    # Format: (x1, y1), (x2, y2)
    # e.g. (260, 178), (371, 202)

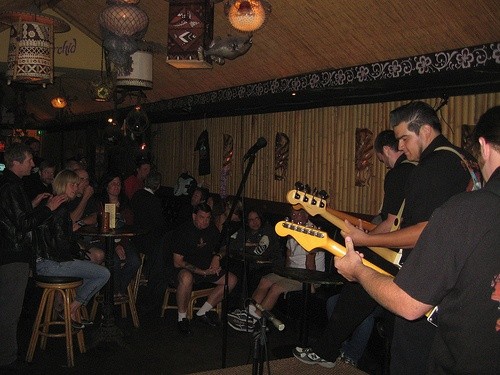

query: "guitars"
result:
(276, 215), (442, 322)
(291, 200), (381, 232)
(287, 181), (406, 270)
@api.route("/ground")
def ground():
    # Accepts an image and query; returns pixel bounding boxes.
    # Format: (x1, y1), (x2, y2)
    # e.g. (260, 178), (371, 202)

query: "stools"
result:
(28, 247), (232, 368)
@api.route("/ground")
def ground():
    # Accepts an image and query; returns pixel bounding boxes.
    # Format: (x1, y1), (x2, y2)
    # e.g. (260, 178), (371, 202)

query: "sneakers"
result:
(176, 317), (191, 334)
(292, 346), (336, 368)
(194, 312), (217, 325)
(227, 308), (260, 332)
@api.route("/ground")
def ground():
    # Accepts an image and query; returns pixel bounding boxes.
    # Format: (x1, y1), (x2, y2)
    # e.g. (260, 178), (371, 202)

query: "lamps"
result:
(0, 0), (269, 108)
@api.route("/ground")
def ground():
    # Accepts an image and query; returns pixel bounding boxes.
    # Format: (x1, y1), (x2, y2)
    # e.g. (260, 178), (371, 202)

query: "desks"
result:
(70, 227), (147, 327)
(273, 266), (343, 360)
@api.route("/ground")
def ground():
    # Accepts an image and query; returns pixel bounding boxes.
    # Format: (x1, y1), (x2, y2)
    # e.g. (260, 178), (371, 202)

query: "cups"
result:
(101, 212), (110, 233)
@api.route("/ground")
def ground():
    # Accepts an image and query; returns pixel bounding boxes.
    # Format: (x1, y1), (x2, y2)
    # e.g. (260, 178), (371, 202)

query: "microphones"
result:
(244, 136), (267, 160)
(251, 298), (285, 332)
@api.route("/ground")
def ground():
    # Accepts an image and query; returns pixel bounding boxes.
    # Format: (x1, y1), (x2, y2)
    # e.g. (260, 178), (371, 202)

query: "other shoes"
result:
(0, 359), (30, 375)
(79, 319), (95, 327)
(57, 309), (85, 328)
(336, 351), (358, 367)
(113, 292), (130, 305)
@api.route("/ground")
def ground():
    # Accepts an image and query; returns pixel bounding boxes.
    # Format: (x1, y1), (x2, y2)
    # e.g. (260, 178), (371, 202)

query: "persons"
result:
(334, 103), (500, 375)
(293, 126), (420, 370)
(0, 141), (375, 375)
(341, 101), (486, 372)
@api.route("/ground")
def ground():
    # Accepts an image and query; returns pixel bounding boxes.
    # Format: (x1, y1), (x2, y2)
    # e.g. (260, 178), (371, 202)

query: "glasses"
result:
(471, 143), (498, 160)
